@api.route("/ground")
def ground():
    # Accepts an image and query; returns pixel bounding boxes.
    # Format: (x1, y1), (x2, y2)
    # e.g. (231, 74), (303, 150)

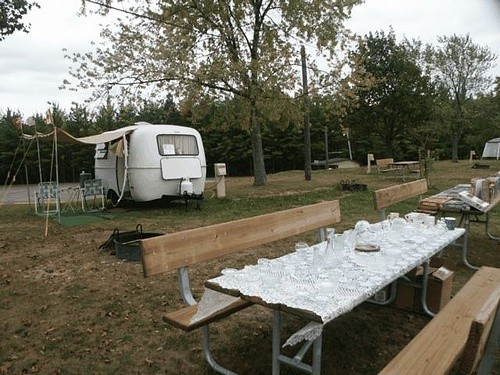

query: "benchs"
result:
(374, 265), (500, 375)
(373, 178), (427, 221)
(376, 158), (435, 183)
(139, 199), (342, 375)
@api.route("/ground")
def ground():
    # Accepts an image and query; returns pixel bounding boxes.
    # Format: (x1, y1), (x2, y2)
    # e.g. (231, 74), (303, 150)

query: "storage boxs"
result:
(373, 267), (453, 314)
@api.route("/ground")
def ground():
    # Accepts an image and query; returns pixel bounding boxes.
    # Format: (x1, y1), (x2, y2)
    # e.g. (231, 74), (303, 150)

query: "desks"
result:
(204, 217), (464, 375)
(389, 161), (419, 181)
(418, 183), (500, 269)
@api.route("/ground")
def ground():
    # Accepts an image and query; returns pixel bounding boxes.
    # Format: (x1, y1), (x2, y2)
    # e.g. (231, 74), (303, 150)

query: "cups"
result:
(219, 213), (457, 294)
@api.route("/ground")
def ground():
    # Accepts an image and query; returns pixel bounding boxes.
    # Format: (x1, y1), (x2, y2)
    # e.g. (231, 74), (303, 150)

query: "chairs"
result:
(33, 179), (106, 216)
